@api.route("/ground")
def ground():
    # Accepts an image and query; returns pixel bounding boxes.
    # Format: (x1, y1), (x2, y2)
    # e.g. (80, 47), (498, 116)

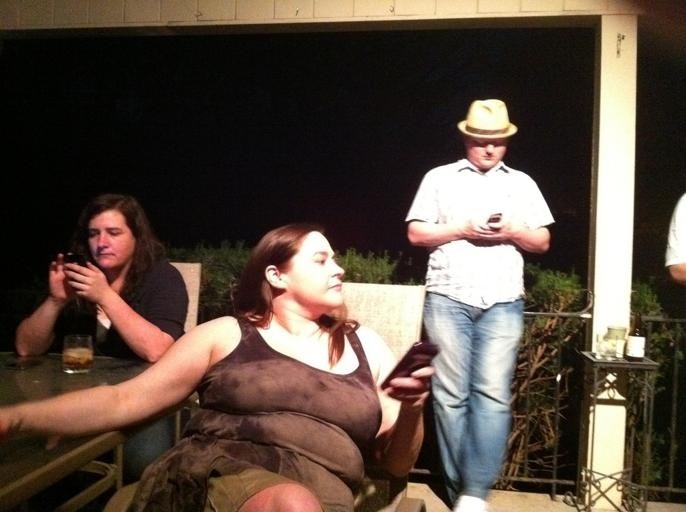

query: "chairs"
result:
(169, 262), (202, 332)
(335, 285), (427, 374)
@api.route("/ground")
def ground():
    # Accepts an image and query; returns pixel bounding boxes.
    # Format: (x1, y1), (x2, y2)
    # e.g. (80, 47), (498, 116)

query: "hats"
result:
(457, 99), (518, 139)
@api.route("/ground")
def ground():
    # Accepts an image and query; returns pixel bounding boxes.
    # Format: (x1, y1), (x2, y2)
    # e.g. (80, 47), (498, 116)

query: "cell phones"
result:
(63, 251), (85, 284)
(486, 212), (502, 224)
(381, 341), (439, 391)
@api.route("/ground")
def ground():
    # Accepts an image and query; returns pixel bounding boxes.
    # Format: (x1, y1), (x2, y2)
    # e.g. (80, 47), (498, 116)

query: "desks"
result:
(0, 351), (187, 512)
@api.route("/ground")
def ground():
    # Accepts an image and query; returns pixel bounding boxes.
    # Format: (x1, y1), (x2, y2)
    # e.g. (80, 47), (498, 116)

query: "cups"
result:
(63, 335), (93, 374)
(596, 328), (625, 359)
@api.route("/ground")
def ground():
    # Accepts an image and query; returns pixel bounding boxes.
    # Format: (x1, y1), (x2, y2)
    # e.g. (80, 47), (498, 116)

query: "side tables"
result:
(563, 350), (658, 511)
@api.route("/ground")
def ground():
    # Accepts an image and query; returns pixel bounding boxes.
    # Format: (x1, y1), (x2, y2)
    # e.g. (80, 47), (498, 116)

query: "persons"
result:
(13, 192), (191, 484)
(665, 193), (686, 289)
(405, 97), (557, 512)
(0, 214), (437, 512)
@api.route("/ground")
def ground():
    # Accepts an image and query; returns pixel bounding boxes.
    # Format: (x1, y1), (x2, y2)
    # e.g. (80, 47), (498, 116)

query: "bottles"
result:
(624, 311), (646, 362)
